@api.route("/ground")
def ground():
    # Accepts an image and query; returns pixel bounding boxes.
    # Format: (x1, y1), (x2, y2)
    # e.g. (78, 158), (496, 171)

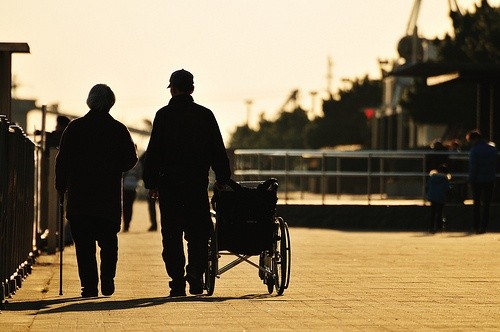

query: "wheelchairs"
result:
(210, 178), (291, 295)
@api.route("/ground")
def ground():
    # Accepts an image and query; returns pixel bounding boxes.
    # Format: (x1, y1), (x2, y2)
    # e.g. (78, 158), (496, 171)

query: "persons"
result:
(122, 145), (139, 232)
(34, 115), (70, 145)
(55, 84), (139, 298)
(425, 167), (450, 233)
(469, 132), (497, 233)
(139, 69), (232, 297)
(140, 148), (159, 231)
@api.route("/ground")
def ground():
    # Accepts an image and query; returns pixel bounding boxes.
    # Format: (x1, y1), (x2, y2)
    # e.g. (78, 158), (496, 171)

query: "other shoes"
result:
(169, 280), (186, 297)
(101, 278), (115, 296)
(185, 265), (204, 295)
(81, 286), (98, 297)
(149, 224), (157, 231)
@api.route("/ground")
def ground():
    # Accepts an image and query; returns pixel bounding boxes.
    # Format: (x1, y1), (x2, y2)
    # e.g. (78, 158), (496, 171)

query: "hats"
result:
(167, 69), (194, 90)
